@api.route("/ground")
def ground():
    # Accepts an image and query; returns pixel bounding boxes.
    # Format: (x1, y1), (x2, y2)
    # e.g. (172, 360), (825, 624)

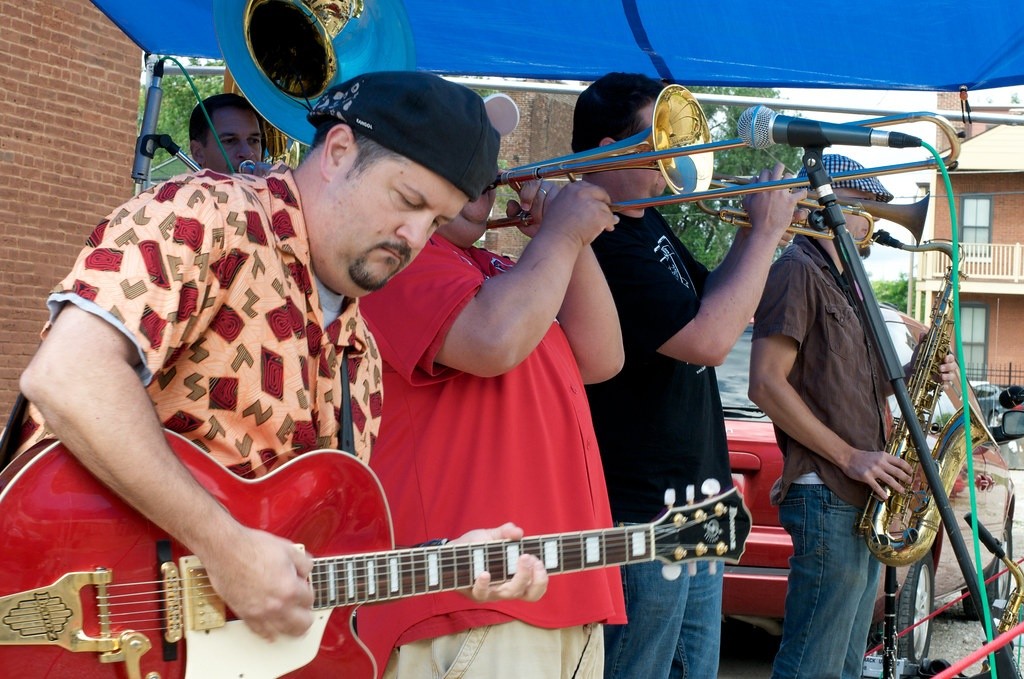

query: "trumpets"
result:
(697, 171), (932, 248)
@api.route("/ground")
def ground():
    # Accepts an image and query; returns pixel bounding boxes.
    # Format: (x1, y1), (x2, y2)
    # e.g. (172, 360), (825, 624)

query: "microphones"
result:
(738, 105), (922, 151)
(239, 160), (257, 175)
(131, 63), (164, 184)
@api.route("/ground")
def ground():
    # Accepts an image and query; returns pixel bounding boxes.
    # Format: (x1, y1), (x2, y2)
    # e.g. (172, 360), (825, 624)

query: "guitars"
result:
(0, 424), (754, 679)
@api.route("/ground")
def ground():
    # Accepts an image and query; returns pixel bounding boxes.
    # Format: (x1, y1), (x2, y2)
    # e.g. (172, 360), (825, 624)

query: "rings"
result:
(539, 187), (547, 195)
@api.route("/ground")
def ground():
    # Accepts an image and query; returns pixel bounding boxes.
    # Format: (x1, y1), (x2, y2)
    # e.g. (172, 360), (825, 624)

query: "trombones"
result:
(487, 83), (962, 230)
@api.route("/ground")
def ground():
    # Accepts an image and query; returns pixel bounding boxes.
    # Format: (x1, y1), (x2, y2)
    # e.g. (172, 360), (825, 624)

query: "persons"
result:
(361, 92), (626, 679)
(573, 73), (809, 679)
(189, 92), (261, 173)
(747, 153), (959, 678)
(20, 70), (549, 638)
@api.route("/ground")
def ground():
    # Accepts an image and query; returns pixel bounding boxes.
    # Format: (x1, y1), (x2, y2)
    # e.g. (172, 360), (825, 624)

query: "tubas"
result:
(208, 0), (416, 174)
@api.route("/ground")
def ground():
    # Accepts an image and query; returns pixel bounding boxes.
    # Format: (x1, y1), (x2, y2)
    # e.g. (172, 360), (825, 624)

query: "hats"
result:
(307, 72), (501, 203)
(481, 94), (520, 137)
(794, 154), (894, 204)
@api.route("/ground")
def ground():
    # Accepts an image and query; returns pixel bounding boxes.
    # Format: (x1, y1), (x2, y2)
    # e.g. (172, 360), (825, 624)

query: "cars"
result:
(712, 299), (1024, 670)
(969, 378), (1002, 429)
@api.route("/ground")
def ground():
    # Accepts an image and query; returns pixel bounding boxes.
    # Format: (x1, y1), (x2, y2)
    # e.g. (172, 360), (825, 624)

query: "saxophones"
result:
(959, 510), (1024, 678)
(854, 228), (990, 567)
